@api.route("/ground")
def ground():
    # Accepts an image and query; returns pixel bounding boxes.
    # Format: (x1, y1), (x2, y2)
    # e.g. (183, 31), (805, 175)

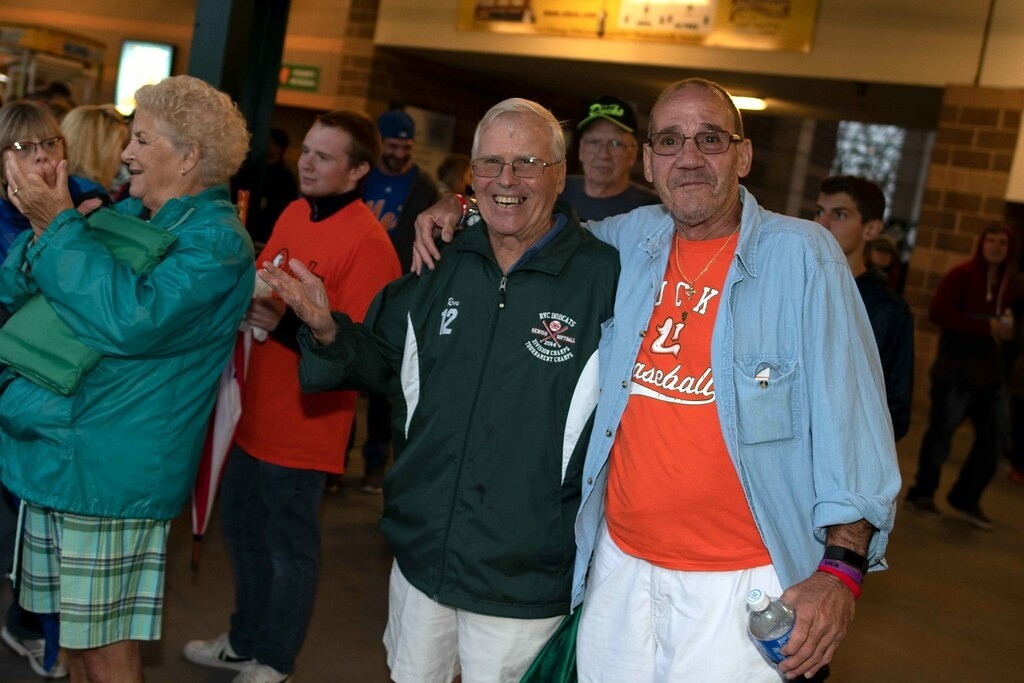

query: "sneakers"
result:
(1, 625), (68, 677)
(182, 632), (255, 669)
(232, 656), (293, 683)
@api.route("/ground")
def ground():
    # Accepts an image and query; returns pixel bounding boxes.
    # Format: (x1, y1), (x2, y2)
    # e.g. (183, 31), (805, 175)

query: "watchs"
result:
(466, 198), (480, 227)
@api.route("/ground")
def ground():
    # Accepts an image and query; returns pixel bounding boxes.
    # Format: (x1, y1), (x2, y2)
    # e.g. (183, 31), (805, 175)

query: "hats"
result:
(377, 110), (415, 139)
(576, 95), (637, 138)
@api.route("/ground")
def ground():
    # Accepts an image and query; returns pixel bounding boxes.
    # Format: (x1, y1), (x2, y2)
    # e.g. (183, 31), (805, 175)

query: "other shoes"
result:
(948, 499), (993, 528)
(903, 498), (938, 517)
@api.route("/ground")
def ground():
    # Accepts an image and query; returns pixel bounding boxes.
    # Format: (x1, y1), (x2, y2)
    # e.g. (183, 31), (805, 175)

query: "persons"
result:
(559, 99), (662, 223)
(182, 112), (401, 683)
(910, 223), (1024, 529)
(255, 96), (619, 683)
(0, 76), (256, 683)
(815, 175), (915, 443)
(863, 234), (895, 282)
(329, 111), (435, 491)
(438, 158), (473, 195)
(252, 128), (294, 242)
(412, 79), (903, 683)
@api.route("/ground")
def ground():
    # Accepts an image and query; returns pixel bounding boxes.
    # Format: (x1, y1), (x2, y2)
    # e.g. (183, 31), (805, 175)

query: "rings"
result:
(13, 188), (19, 195)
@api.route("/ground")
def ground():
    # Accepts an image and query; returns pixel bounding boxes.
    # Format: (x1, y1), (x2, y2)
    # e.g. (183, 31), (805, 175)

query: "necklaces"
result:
(676, 224), (741, 300)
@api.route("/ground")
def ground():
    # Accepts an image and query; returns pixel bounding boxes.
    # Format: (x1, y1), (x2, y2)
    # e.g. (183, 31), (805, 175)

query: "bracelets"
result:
(817, 566), (861, 602)
(820, 560), (863, 589)
(454, 194), (468, 226)
(822, 545), (868, 575)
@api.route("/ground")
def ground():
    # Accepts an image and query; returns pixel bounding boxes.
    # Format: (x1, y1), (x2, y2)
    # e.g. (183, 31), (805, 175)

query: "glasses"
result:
(650, 131), (741, 156)
(582, 139), (638, 156)
(4, 136), (63, 157)
(470, 157), (560, 178)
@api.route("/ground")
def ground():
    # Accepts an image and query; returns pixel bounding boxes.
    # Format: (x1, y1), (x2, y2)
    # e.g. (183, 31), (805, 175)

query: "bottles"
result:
(1000, 309), (1013, 339)
(747, 590), (830, 683)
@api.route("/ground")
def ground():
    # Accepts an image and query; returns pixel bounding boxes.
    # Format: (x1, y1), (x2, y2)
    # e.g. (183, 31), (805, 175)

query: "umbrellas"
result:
(187, 325), (252, 569)
